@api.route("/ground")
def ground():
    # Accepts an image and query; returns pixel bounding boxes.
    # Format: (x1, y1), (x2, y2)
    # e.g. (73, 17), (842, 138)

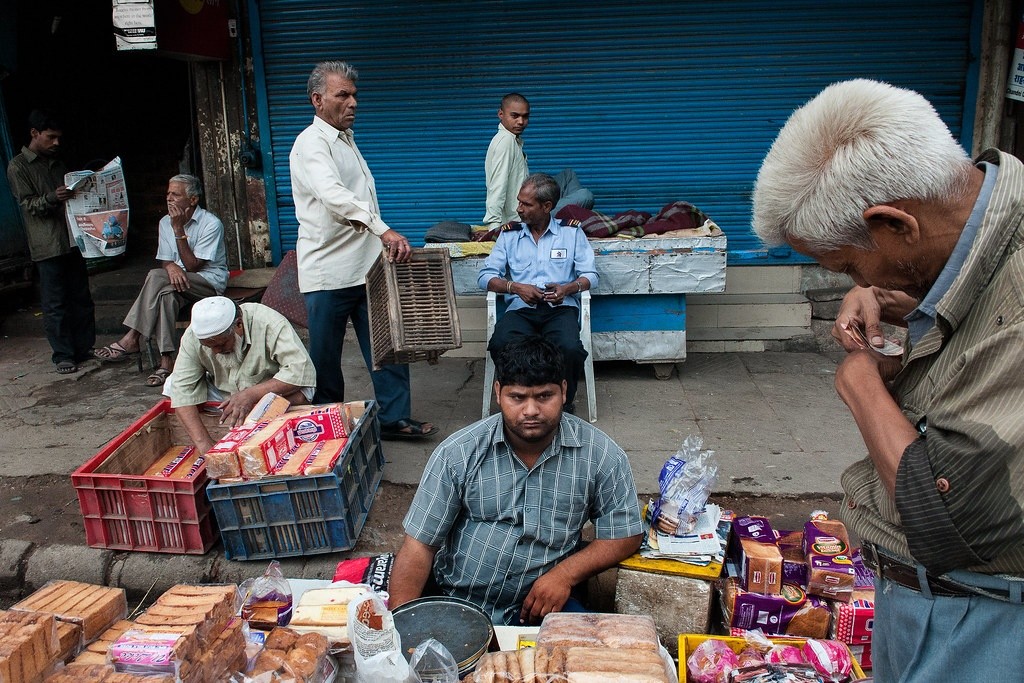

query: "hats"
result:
(190, 295), (235, 339)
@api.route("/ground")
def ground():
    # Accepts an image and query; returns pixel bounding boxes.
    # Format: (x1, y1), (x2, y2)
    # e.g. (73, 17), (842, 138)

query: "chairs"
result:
(481, 291), (598, 424)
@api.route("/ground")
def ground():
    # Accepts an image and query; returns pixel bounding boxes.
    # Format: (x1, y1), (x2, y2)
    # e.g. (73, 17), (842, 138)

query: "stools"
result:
(263, 249), (309, 330)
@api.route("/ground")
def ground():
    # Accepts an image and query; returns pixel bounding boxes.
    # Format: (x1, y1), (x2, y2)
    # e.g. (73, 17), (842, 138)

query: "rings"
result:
(554, 293), (558, 297)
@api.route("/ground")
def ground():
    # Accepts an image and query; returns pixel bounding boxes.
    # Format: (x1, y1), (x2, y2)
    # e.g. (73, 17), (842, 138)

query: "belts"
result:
(859, 540), (1024, 605)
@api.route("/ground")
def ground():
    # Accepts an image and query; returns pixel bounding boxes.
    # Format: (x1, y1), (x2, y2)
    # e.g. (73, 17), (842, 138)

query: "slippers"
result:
(380, 419), (440, 440)
(93, 343), (142, 363)
(57, 360), (76, 374)
(145, 368), (171, 386)
(77, 349), (96, 360)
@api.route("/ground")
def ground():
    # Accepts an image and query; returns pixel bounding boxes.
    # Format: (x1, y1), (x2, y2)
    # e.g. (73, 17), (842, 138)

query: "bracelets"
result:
(574, 280), (584, 292)
(507, 281), (513, 295)
(175, 235), (187, 239)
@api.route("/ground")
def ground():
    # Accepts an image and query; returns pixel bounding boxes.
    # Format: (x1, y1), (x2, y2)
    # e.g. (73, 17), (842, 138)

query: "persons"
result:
(750, 79), (1024, 683)
(289, 62), (441, 439)
(162, 296), (316, 457)
(483, 94), (594, 233)
(7, 106), (95, 374)
(93, 175), (230, 387)
(477, 172), (599, 415)
(388, 335), (645, 626)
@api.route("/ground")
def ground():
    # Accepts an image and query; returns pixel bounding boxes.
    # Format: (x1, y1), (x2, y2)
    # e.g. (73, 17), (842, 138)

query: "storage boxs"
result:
(677, 630), (868, 683)
(71, 397), (385, 563)
(365, 249), (463, 370)
(613, 567), (715, 652)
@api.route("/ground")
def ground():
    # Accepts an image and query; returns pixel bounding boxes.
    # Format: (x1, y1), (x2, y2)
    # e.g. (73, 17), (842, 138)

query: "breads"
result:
(720, 515), (856, 641)
(0, 579), (371, 683)
(204, 391), (352, 483)
(462, 611), (670, 683)
(140, 444), (202, 482)
